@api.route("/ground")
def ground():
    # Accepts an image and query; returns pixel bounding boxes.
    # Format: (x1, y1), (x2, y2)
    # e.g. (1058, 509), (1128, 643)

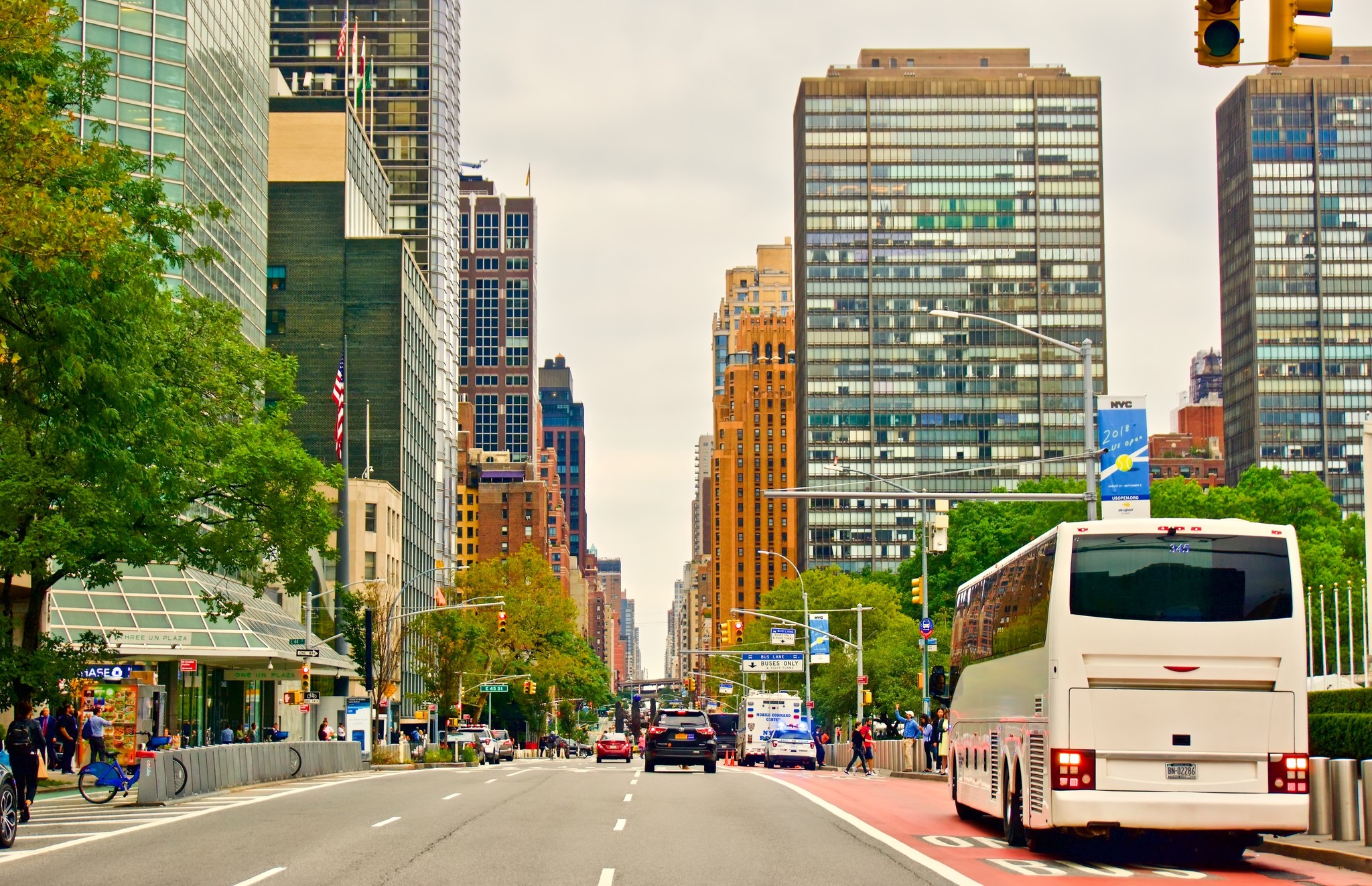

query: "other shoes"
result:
(941, 772), (945, 775)
(902, 769), (912, 772)
(23, 803), (30, 822)
(549, 756), (550, 758)
(52, 763), (58, 771)
(70, 770), (78, 774)
(18, 812), (24, 823)
(819, 763), (826, 766)
(640, 756), (643, 759)
(62, 771), (71, 774)
(630, 757), (633, 759)
(554, 751), (555, 754)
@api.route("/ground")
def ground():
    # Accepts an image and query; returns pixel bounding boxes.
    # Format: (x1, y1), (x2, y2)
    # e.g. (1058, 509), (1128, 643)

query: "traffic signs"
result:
(296, 649), (319, 658)
(707, 702), (717, 710)
(421, 700), (436, 706)
(741, 627), (804, 674)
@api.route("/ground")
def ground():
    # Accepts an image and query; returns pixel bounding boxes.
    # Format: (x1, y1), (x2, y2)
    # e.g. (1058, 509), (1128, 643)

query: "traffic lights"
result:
(556, 711), (561, 716)
(613, 693), (618, 698)
(523, 680), (536, 695)
(302, 666), (310, 687)
(589, 702), (593, 711)
(461, 687), (465, 698)
(695, 701), (702, 710)
(911, 575), (923, 605)
(1267, 0), (1333, 67)
(1193, 0), (1244, 65)
(719, 619), (743, 644)
(283, 691), (300, 703)
(684, 677), (697, 692)
(498, 611), (507, 634)
(447, 719), (453, 726)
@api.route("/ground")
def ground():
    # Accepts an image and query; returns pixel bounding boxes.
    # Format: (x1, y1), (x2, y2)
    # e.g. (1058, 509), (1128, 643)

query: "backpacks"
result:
(410, 731), (418, 742)
(81, 719), (92, 740)
(636, 736), (642, 744)
(7, 717), (32, 750)
(548, 736), (554, 743)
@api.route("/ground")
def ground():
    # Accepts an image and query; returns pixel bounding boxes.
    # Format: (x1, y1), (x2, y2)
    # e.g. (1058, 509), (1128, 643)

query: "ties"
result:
(43, 717), (47, 736)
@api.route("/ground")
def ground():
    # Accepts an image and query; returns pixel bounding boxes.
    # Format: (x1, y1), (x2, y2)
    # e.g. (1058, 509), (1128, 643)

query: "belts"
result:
(903, 737), (914, 739)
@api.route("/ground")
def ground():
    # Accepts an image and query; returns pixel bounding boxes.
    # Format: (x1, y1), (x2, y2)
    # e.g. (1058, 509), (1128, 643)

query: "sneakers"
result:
(935, 769), (940, 773)
(844, 766), (878, 776)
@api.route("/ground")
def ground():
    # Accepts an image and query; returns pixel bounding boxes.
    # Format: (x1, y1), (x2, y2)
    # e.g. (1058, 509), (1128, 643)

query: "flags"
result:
(331, 349), (344, 461)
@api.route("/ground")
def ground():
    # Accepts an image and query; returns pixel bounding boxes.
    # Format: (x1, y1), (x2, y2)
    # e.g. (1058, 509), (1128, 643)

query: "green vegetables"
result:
(115, 705), (124, 722)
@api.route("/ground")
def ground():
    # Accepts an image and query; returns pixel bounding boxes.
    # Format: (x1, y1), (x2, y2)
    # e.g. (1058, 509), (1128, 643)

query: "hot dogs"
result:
(125, 736), (133, 742)
(104, 714), (114, 718)
(125, 714), (133, 720)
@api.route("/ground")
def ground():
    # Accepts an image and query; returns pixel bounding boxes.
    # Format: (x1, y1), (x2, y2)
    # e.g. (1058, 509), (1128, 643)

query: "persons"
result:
(164, 728), (173, 747)
(419, 730), (427, 743)
(895, 704), (949, 775)
(184, 719), (196, 745)
(539, 731), (557, 758)
(812, 724), (829, 766)
(318, 723), (331, 741)
(249, 723), (258, 743)
(845, 717), (884, 777)
(932, 675), (945, 695)
(5, 700), (46, 823)
(33, 703), (79, 775)
(411, 727), (420, 744)
(221, 724), (233, 744)
(337, 723), (346, 741)
(234, 723), (249, 744)
(603, 730), (645, 759)
(88, 707), (117, 763)
(373, 727), (381, 745)
(264, 723), (281, 742)
(448, 727), (457, 733)
(403, 734), (410, 742)
(395, 728), (401, 744)
(323, 720), (335, 741)
(385, 727), (396, 744)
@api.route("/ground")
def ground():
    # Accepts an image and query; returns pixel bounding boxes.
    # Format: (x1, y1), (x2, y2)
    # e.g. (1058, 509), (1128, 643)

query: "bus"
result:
(927, 516), (1310, 864)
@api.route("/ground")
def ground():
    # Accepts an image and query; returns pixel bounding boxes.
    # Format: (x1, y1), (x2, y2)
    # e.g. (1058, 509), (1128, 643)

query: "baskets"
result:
(146, 736), (172, 748)
(274, 732), (289, 740)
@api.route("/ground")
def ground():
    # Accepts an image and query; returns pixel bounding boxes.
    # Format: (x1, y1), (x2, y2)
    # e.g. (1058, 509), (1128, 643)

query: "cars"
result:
(434, 684), (818, 774)
(0, 761), (19, 848)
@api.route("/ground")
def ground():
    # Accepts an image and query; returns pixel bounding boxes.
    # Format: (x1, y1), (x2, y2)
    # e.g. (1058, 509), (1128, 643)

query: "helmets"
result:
(550, 731), (555, 734)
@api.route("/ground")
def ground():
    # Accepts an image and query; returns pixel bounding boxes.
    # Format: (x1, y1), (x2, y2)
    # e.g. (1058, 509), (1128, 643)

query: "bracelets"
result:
(850, 748), (852, 748)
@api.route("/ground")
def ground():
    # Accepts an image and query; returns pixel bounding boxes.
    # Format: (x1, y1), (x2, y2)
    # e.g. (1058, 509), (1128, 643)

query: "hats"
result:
(452, 727), (457, 730)
(905, 711), (914, 717)
(338, 722), (344, 725)
(814, 725), (821, 729)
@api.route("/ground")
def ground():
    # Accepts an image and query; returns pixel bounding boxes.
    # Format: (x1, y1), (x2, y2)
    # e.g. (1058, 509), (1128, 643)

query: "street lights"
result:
(384, 565), (470, 743)
(432, 595), (505, 743)
(928, 309), (1098, 520)
(708, 654), (748, 697)
(303, 578), (389, 741)
(755, 465), (930, 742)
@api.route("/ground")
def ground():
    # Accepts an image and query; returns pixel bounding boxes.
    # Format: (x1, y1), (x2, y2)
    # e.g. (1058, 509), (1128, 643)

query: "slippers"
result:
(921, 770), (932, 772)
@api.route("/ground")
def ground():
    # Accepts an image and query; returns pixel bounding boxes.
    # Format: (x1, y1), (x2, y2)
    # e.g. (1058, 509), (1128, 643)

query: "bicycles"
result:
(76, 730), (189, 805)
